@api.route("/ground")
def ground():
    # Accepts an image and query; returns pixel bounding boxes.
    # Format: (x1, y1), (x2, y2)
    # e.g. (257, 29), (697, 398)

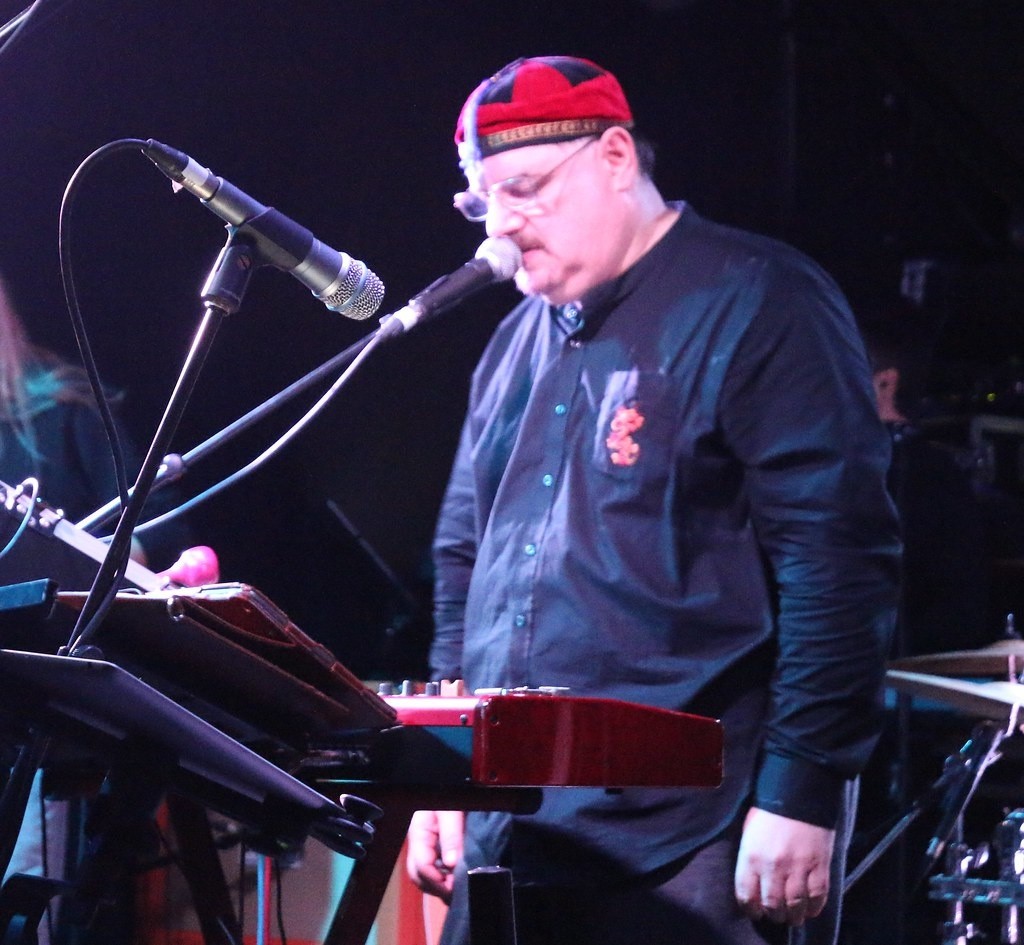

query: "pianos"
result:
(0, 668), (728, 944)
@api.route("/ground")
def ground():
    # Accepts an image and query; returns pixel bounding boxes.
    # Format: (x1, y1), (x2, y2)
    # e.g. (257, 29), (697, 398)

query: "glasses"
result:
(451, 136), (601, 221)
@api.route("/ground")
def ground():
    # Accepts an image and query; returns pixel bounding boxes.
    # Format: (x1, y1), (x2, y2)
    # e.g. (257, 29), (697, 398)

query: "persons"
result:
(404, 55), (893, 945)
(0, 274), (193, 943)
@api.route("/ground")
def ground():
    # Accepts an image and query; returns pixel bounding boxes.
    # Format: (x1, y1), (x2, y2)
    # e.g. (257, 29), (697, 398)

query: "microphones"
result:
(141, 140), (386, 320)
(374, 235), (524, 343)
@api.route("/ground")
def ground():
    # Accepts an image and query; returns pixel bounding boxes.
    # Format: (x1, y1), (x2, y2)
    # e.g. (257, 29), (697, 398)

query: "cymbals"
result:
(884, 636), (1023, 732)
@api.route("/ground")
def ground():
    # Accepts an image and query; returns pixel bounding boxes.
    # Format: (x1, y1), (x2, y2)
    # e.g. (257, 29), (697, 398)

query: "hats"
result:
(458, 52), (637, 167)
